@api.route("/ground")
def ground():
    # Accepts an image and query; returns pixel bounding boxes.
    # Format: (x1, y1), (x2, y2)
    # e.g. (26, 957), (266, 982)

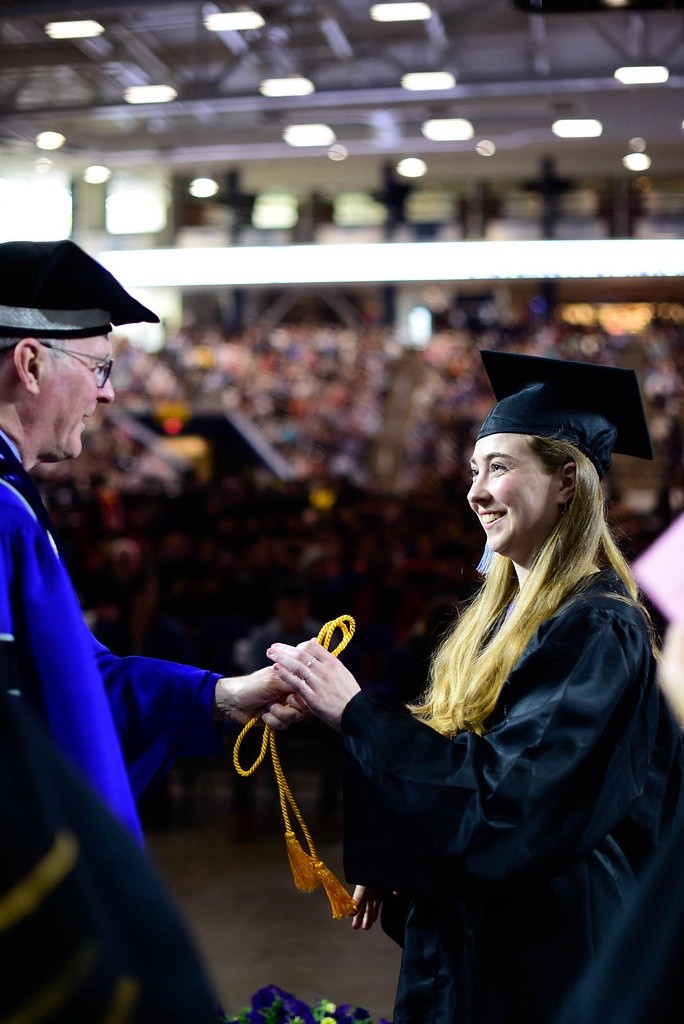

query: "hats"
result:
(0, 239), (161, 340)
(476, 350), (654, 577)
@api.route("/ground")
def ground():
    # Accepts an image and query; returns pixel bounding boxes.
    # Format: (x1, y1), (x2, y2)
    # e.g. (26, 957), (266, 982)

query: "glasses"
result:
(4, 341), (114, 388)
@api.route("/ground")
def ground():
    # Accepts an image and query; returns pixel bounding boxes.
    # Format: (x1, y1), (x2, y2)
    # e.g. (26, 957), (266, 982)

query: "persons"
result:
(514, 156), (578, 236)
(370, 163), (414, 241)
(156, 165), (254, 247)
(457, 177), (504, 240)
(288, 191), (333, 245)
(598, 173), (646, 238)
(0, 237), (684, 1024)
(265, 349), (684, 1024)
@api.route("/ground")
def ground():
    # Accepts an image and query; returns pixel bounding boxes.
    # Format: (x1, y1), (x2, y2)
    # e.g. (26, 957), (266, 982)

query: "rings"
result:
(303, 671), (313, 680)
(306, 657), (316, 667)
